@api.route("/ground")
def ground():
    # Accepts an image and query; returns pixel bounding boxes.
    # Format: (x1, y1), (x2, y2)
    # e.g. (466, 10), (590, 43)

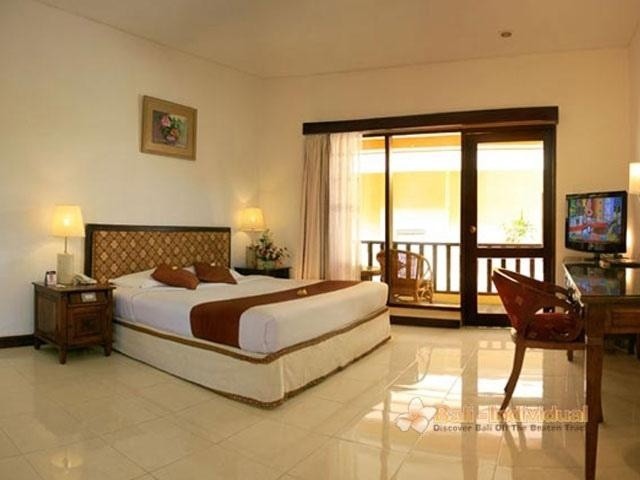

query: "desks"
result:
(564, 263), (640, 480)
(359, 266), (383, 281)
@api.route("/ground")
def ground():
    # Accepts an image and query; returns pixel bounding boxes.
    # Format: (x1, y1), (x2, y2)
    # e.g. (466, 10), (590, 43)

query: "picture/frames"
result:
(141, 94), (198, 161)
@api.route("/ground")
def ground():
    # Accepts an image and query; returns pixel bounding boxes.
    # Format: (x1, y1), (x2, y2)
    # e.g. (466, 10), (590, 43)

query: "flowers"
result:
(255, 229), (291, 262)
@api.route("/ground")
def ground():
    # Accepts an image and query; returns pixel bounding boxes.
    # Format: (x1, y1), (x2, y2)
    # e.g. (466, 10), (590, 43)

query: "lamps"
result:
(49, 204), (86, 285)
(239, 207), (266, 268)
(628, 162), (640, 196)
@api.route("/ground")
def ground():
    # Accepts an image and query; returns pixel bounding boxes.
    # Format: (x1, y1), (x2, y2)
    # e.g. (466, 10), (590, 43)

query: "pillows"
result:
(150, 263), (200, 290)
(189, 265), (244, 281)
(108, 266), (191, 288)
(192, 260), (238, 284)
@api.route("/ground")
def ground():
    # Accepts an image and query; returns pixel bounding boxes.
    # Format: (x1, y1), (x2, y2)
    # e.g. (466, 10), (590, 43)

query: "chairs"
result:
(376, 249), (434, 307)
(491, 266), (605, 425)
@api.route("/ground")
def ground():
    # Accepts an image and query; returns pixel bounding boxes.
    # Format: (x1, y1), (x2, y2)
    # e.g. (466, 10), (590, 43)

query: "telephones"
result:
(74, 271), (97, 284)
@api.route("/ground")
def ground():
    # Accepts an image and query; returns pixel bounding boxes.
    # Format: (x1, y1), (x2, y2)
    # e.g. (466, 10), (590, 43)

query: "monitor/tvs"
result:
(565, 190), (628, 260)
(563, 260), (625, 296)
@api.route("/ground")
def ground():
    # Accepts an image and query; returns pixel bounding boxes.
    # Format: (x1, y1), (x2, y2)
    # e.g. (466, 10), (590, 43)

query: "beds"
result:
(84, 223), (393, 411)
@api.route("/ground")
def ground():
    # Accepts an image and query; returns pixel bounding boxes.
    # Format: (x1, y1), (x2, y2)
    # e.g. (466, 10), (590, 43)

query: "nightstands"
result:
(31, 280), (118, 365)
(234, 267), (292, 279)
